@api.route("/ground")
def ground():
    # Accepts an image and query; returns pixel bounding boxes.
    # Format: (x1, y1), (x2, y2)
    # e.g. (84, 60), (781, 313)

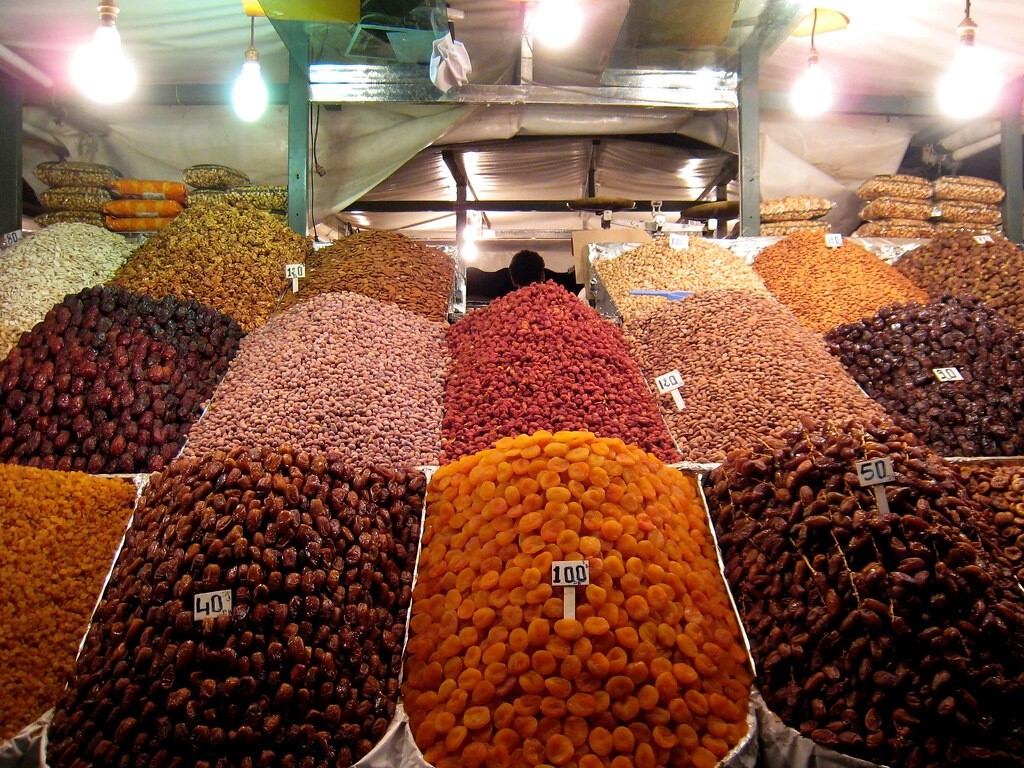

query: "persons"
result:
(509, 249), (545, 289)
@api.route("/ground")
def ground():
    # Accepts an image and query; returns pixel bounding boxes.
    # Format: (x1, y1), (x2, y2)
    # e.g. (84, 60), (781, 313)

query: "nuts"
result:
(165, 225), (1024, 594)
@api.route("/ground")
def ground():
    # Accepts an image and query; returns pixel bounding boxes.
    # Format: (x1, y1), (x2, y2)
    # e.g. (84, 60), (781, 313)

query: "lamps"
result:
(937, 0), (999, 118)
(790, 7), (834, 116)
(231, 17), (270, 124)
(74, 0), (139, 106)
